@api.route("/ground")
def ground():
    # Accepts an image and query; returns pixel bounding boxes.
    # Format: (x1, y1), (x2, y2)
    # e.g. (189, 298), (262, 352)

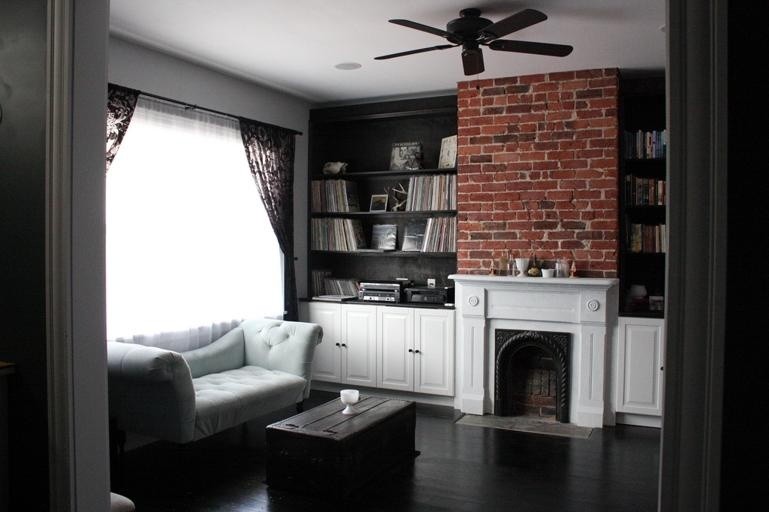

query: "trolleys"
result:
(373, 8), (573, 76)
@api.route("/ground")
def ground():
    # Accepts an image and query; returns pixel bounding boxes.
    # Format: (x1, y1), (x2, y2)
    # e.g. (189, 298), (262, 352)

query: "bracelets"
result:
(541, 269), (555, 278)
(631, 285), (647, 300)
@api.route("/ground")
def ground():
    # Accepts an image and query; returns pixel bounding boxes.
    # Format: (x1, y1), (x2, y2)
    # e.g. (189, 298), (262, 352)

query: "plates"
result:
(611, 69), (663, 431)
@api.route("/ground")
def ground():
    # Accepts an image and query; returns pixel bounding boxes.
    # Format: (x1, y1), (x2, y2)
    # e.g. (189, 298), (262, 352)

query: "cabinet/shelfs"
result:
(499, 249), (509, 276)
(611, 69), (663, 431)
(298, 94), (458, 410)
(506, 254), (515, 276)
(570, 261), (577, 278)
(555, 256), (570, 279)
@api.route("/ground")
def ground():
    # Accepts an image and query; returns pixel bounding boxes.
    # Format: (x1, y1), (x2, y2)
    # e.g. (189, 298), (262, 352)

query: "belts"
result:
(340, 389), (360, 414)
(515, 258), (529, 277)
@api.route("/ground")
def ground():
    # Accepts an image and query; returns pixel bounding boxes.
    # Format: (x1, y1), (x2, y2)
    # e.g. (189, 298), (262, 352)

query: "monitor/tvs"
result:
(309, 172), (457, 253)
(310, 270), (361, 299)
(622, 127), (666, 253)
(437, 134), (457, 169)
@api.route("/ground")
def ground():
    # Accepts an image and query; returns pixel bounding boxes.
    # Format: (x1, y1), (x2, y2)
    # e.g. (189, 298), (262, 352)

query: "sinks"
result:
(106, 315), (324, 453)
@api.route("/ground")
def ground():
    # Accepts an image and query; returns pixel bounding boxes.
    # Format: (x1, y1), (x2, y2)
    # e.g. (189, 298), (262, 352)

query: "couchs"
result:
(106, 315), (324, 453)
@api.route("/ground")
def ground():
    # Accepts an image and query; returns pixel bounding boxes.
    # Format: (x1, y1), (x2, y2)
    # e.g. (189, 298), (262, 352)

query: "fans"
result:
(373, 8), (573, 76)
